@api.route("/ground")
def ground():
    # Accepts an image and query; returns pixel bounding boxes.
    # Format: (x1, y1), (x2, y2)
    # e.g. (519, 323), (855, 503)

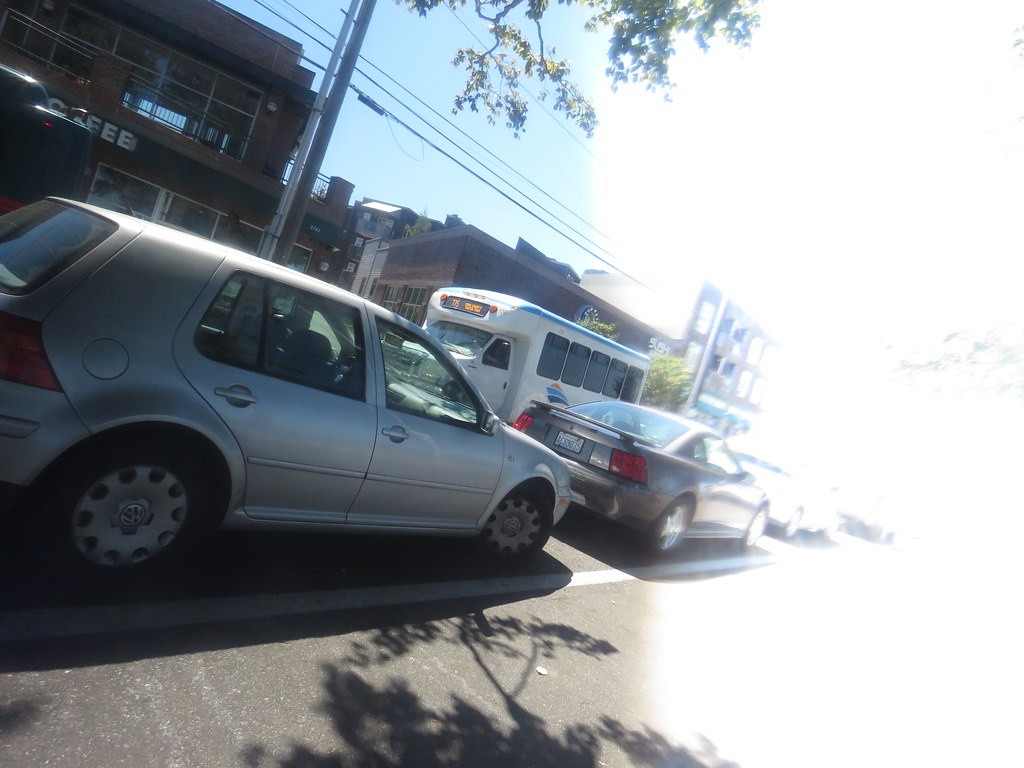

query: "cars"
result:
(707, 434), (836, 538)
(511, 399), (771, 555)
(840, 491), (896, 542)
(381, 332), (403, 357)
(0, 196), (574, 579)
(205, 294), (287, 341)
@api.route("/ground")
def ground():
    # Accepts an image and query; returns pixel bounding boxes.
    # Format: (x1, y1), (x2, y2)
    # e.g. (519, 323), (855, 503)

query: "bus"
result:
(0, 64), (93, 215)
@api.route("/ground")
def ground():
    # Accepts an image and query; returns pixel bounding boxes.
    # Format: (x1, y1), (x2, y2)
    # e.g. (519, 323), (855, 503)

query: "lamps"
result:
(316, 260), (329, 275)
(265, 96), (280, 116)
(41, 0), (57, 17)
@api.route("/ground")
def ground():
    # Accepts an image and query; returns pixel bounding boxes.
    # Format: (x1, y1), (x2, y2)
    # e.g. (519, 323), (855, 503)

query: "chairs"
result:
(612, 421), (632, 432)
(246, 313), (332, 389)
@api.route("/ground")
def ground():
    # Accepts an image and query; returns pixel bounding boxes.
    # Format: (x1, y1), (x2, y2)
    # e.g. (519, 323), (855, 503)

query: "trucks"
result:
(395, 287), (651, 425)
